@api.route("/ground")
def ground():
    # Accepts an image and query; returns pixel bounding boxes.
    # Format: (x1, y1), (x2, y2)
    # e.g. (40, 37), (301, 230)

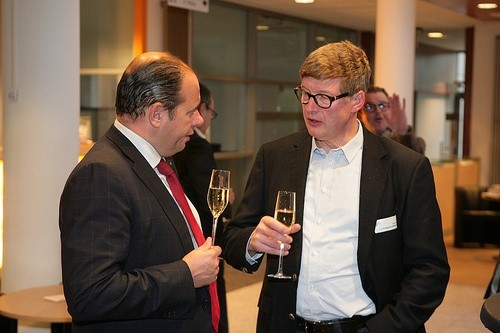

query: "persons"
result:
(222, 39), (452, 332)
(173, 84), (235, 333)
(58, 50), (224, 333)
(360, 87), (426, 157)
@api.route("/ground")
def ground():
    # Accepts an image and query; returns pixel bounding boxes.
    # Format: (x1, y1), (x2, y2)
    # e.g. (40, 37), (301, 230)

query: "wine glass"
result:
(268, 191), (296, 278)
(207, 169), (230, 249)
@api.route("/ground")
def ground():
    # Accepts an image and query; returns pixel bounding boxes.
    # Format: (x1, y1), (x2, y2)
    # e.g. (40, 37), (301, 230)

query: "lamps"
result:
(416, 24), (446, 41)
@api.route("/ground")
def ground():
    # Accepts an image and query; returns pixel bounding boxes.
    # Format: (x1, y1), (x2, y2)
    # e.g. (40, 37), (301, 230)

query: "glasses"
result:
(365, 102), (390, 112)
(206, 106), (217, 120)
(294, 85), (349, 109)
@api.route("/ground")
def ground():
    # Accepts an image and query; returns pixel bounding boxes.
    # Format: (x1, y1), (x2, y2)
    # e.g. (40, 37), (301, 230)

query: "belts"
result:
(296, 316), (364, 333)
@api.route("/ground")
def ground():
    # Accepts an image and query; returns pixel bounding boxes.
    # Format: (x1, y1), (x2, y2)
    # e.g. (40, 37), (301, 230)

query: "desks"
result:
(0, 284), (73, 333)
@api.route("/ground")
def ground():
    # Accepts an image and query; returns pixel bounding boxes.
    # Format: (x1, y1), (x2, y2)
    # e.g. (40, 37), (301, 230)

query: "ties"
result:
(157, 160), (220, 333)
